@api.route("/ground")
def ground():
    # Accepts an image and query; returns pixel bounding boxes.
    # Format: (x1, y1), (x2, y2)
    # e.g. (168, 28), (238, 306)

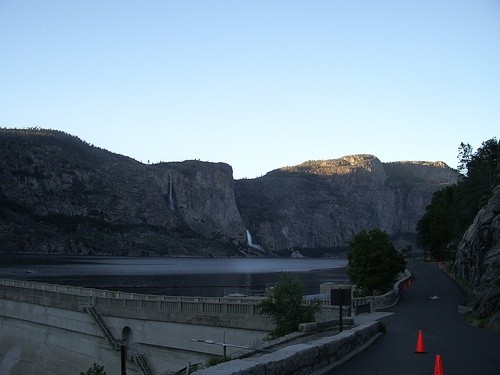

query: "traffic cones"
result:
(433, 355), (445, 375)
(414, 329), (428, 353)
